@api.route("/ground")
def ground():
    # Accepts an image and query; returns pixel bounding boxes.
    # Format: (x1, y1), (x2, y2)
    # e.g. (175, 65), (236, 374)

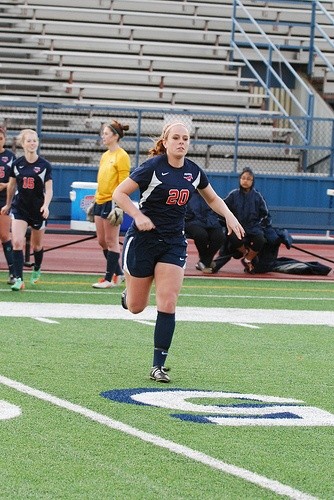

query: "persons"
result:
(86, 121), (131, 289)
(203, 167), (271, 274)
(1, 129), (53, 291)
(112, 121), (245, 382)
(184, 185), (225, 270)
(0, 128), (17, 285)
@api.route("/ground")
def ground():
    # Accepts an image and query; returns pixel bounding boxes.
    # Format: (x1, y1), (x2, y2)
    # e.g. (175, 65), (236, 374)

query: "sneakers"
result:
(7, 275), (15, 285)
(150, 365), (171, 383)
(241, 258), (256, 275)
(30, 266), (40, 286)
(92, 278), (113, 288)
(196, 261), (216, 271)
(111, 273), (125, 287)
(121, 288), (128, 310)
(197, 260), (212, 274)
(11, 278), (24, 291)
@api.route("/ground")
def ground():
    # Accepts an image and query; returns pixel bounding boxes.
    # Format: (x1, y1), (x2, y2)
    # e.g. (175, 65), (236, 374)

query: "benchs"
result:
(0, 0), (334, 268)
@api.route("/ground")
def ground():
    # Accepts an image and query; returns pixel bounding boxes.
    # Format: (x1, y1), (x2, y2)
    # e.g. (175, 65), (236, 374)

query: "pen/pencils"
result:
(70, 182), (98, 230)
